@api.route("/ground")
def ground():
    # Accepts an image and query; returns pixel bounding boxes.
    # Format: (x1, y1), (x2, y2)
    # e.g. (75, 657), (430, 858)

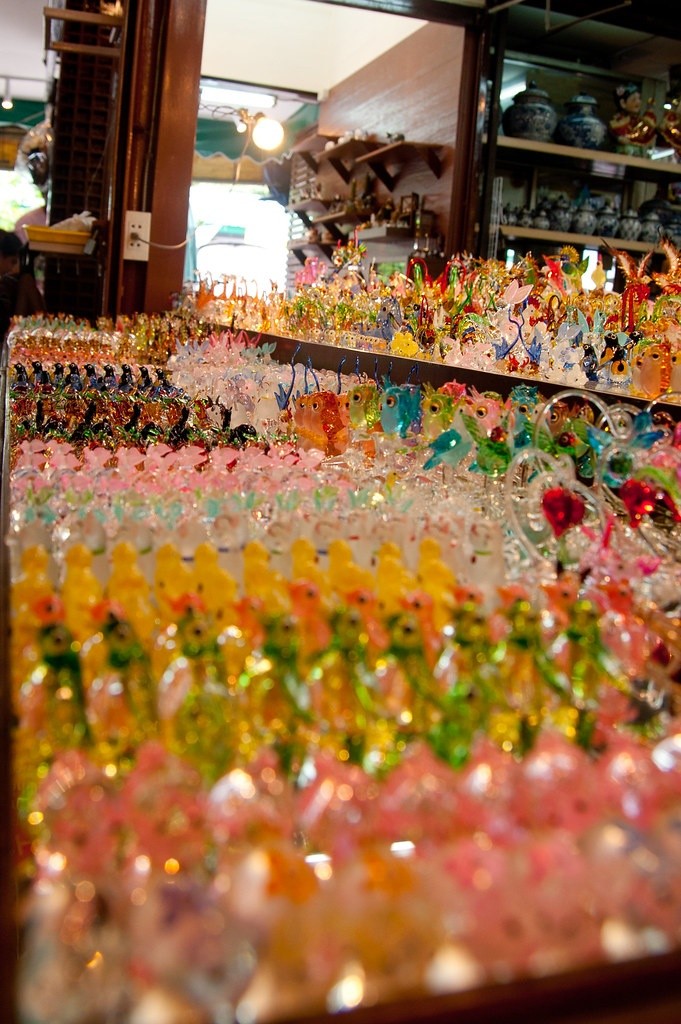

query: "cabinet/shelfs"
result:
(484, 133), (681, 286)
(288, 130), (443, 266)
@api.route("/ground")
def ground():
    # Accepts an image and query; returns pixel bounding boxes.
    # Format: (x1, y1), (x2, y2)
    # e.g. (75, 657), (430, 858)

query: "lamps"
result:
(239, 108), (285, 152)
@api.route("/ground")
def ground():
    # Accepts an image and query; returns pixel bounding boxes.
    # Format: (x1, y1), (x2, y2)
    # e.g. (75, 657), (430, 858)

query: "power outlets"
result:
(124, 211), (150, 261)
